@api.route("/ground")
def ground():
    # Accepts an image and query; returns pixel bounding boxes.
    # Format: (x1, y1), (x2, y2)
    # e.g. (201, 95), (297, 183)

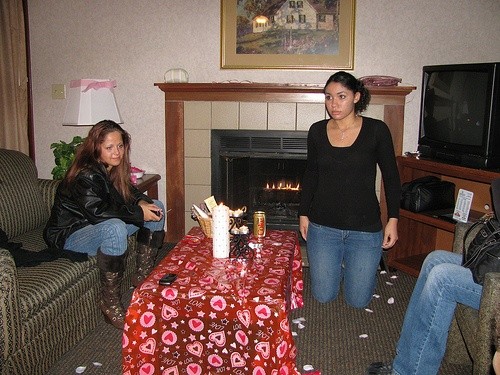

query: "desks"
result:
(133, 174), (161, 200)
(122, 227), (303, 375)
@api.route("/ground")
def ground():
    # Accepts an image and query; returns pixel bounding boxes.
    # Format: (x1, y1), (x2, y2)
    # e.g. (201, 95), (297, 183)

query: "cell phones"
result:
(159, 273), (177, 285)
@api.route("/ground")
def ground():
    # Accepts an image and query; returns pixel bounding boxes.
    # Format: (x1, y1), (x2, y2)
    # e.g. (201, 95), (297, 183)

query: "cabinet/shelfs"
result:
(385, 154), (500, 281)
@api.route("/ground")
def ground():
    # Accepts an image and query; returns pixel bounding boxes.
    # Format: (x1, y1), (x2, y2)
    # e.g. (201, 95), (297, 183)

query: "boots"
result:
(132, 227), (165, 288)
(96, 246), (126, 330)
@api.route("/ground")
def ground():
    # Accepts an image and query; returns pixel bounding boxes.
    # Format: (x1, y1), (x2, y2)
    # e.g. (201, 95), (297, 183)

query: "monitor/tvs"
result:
(419, 61), (500, 172)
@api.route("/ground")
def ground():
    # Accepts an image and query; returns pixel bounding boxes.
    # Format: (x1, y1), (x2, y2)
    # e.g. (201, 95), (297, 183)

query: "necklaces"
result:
(340, 132), (345, 140)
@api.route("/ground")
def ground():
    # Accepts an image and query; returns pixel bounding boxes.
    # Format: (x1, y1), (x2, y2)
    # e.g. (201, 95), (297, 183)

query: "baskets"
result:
(197, 215), (236, 238)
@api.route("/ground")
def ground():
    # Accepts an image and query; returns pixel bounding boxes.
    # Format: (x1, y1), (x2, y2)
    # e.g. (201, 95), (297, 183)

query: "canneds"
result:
(253, 211), (266, 237)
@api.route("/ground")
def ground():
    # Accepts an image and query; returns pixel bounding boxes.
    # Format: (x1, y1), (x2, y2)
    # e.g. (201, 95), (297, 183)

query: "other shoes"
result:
(366, 361), (393, 375)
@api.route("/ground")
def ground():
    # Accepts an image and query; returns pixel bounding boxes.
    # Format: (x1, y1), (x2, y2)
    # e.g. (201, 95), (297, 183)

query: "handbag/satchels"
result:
(401, 175), (455, 216)
(461, 217), (500, 286)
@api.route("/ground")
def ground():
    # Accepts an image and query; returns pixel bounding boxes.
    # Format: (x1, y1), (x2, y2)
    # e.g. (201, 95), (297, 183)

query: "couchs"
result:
(0, 147), (138, 375)
(446, 215), (500, 374)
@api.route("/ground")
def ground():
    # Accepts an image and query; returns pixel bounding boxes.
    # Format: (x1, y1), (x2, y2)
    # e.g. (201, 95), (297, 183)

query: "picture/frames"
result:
(220, 0), (356, 72)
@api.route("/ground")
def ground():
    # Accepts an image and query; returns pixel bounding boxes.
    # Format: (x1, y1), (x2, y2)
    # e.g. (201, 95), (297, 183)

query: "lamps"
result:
(63, 79), (124, 127)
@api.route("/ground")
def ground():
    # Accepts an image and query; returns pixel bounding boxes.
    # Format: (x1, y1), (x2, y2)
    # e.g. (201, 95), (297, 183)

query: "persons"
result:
(365, 176), (500, 375)
(299, 71), (402, 309)
(42, 120), (166, 333)
